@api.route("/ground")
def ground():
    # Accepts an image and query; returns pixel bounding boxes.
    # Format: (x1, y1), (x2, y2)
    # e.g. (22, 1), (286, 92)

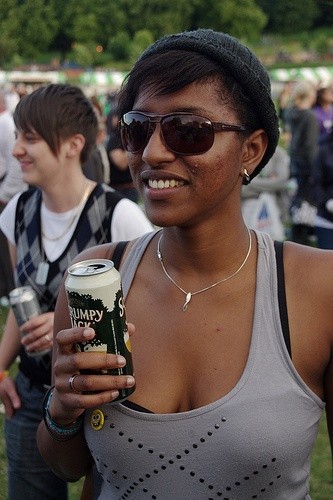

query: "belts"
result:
(19, 365), (52, 394)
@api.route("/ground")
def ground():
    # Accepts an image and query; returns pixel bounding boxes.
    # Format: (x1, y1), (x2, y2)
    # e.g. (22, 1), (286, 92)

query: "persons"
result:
(0, 85), (156, 500)
(35, 29), (333, 500)
(0, 79), (333, 251)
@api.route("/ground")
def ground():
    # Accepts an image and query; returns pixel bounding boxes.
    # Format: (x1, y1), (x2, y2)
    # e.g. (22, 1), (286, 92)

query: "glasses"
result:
(117, 109), (248, 156)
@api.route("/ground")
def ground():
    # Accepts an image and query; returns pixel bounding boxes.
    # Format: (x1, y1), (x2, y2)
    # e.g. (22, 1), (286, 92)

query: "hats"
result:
(132, 27), (281, 185)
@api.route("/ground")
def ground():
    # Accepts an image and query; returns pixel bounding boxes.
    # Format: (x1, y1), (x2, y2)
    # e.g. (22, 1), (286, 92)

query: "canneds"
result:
(65, 258), (136, 406)
(8, 285), (52, 356)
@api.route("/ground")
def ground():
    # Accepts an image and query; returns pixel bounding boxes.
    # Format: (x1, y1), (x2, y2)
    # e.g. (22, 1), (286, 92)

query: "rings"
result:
(45, 335), (53, 344)
(69, 374), (81, 394)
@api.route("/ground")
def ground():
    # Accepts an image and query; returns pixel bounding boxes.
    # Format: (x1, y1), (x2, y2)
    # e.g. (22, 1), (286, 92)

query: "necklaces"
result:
(40, 181), (89, 242)
(158, 227), (251, 313)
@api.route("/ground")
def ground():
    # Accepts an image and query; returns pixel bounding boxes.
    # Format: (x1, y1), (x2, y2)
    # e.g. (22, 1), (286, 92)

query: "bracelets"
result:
(0, 370), (9, 381)
(42, 386), (84, 442)
